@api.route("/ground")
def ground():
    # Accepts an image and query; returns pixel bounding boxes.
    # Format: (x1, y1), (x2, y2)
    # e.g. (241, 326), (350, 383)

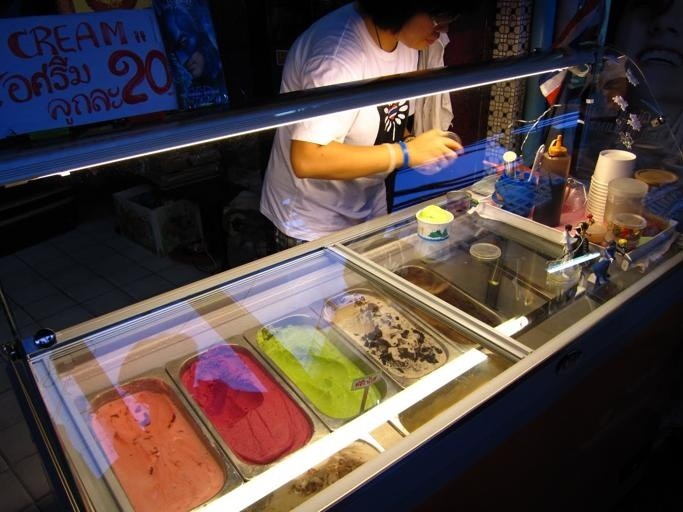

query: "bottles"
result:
(532, 134), (571, 227)
(603, 177), (650, 232)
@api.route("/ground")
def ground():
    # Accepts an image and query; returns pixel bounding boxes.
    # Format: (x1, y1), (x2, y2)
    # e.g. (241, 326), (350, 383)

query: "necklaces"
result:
(370, 17), (382, 47)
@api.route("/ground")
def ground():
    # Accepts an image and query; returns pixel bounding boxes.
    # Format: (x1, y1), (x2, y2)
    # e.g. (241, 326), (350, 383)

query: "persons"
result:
(259, 0), (464, 255)
(610, 1), (681, 108)
(573, 220), (588, 272)
(559, 223), (579, 279)
(159, 4), (226, 108)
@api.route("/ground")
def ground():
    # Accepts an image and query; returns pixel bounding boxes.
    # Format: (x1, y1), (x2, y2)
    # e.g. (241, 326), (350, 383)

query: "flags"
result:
(535, 0), (606, 107)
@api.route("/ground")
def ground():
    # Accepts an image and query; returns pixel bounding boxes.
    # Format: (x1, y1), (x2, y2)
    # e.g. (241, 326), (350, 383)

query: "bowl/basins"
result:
(414, 206), (455, 243)
(584, 148), (639, 224)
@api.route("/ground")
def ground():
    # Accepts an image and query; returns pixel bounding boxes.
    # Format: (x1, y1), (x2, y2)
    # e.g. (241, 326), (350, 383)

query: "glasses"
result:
(424, 14), (455, 33)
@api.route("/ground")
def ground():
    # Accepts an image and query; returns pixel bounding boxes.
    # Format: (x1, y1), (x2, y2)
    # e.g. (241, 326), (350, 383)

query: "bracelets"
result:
(380, 141), (396, 175)
(397, 139), (408, 167)
